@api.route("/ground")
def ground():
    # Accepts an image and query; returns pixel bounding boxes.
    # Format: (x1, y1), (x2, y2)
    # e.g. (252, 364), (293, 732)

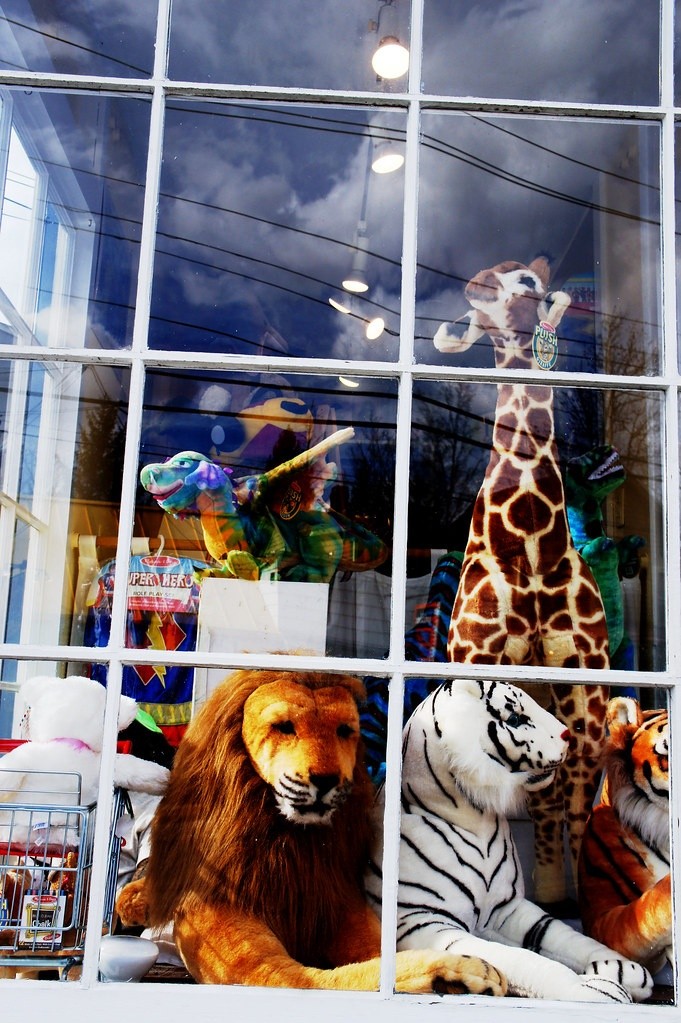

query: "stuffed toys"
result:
(361, 678), (656, 1006)
(580, 698), (670, 959)
(0, 673), (172, 847)
(140, 375), (644, 653)
(433, 254), (612, 904)
(117, 647), (508, 996)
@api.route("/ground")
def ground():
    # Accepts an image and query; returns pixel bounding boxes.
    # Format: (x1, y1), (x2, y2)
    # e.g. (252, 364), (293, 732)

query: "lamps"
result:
(365, 111), (404, 174)
(338, 377), (361, 388)
(371, 4), (410, 79)
(328, 237), (386, 339)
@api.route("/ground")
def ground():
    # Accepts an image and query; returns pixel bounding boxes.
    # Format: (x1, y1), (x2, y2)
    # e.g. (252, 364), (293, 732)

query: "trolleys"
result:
(0, 769), (127, 980)
